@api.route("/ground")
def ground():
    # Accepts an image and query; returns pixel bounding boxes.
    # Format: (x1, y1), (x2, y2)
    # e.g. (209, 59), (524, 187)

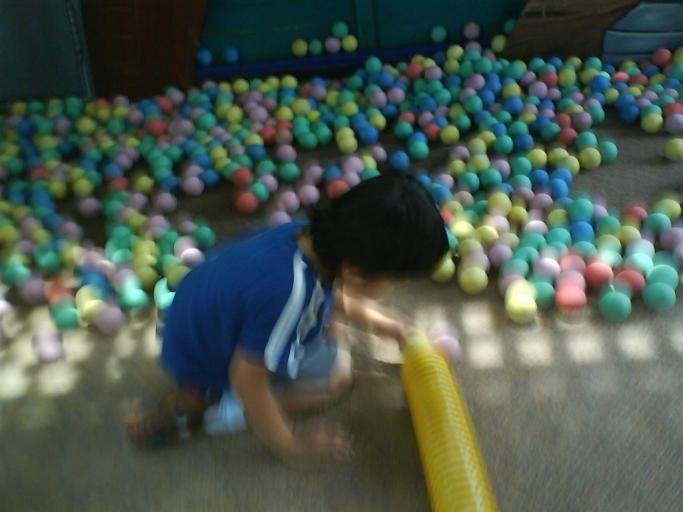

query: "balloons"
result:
(396, 327), (498, 512)
(456, 266), (489, 295)
(11, 100), (27, 116)
(0, 225), (21, 246)
(16, 239), (33, 255)
(75, 285), (101, 306)
(122, 287), (149, 311)
(30, 332), (62, 360)
(645, 265), (678, 291)
(18, 277), (41, 305)
(54, 306), (79, 325)
(78, 298), (107, 326)
(2, 252), (29, 270)
(38, 248), (60, 274)
(26, 99), (44, 112)
(641, 282), (676, 314)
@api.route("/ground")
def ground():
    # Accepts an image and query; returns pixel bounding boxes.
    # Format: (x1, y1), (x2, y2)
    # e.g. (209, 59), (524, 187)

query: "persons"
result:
(122, 169), (453, 465)
